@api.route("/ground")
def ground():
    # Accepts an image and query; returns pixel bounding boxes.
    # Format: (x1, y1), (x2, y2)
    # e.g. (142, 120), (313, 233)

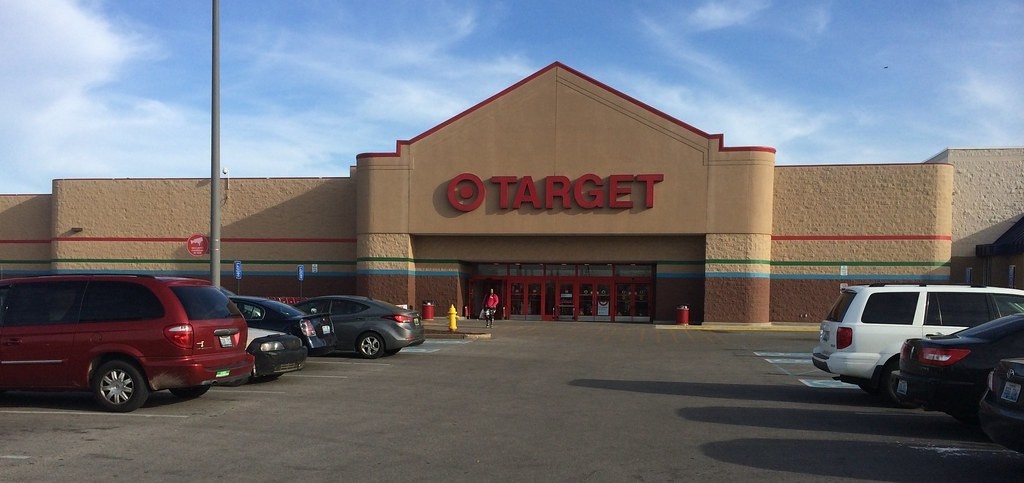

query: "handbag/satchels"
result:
(478, 308), (486, 319)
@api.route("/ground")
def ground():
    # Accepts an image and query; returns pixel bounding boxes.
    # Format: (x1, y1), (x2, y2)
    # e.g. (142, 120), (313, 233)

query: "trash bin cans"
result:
(422, 300), (435, 320)
(676, 304), (690, 326)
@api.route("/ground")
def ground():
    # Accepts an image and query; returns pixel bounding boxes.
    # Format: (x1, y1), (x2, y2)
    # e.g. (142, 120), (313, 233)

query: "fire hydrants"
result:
(446, 304), (461, 333)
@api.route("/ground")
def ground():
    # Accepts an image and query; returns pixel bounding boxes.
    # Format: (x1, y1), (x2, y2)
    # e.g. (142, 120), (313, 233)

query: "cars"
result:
(290, 294), (427, 359)
(890, 311), (1023, 419)
(226, 293), (338, 353)
(975, 357), (1023, 455)
(247, 323), (308, 382)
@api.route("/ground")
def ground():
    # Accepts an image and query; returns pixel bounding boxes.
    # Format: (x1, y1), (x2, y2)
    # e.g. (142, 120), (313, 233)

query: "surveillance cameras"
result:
(223, 170), (228, 174)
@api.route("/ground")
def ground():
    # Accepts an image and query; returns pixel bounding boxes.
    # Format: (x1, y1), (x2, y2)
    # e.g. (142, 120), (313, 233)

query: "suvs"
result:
(810, 280), (1023, 404)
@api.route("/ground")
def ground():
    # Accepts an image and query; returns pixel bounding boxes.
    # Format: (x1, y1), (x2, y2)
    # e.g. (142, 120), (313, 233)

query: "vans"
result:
(0, 273), (255, 412)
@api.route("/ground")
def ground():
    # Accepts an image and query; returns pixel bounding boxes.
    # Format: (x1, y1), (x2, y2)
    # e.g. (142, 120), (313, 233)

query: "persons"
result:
(480, 288), (498, 328)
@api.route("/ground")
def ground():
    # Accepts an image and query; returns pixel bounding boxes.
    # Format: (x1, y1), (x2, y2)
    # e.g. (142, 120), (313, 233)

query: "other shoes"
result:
(486, 325), (490, 328)
(490, 325), (493, 328)
